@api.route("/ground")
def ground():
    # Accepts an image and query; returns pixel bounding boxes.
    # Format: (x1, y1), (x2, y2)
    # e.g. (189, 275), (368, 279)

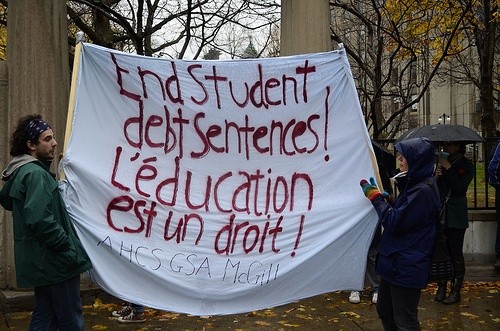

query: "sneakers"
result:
(117, 310), (147, 323)
(112, 305), (133, 317)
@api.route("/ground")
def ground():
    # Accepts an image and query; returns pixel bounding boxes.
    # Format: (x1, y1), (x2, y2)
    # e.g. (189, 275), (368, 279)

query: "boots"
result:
(435, 275), (448, 302)
(443, 274), (464, 305)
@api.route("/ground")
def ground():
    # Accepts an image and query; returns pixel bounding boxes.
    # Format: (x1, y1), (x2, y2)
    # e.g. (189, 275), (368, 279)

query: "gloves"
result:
(359, 177), (383, 202)
(381, 192), (394, 206)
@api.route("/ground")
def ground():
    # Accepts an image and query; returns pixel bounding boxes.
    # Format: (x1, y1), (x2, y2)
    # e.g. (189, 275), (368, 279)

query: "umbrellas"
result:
(396, 123), (484, 143)
(372, 140), (396, 178)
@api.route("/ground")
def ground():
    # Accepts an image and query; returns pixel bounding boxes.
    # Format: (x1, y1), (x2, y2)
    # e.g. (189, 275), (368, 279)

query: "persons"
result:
(111, 302), (146, 323)
(434, 142), (475, 305)
(0, 115), (92, 331)
(349, 152), (383, 304)
(488, 142), (500, 279)
(359, 136), (442, 331)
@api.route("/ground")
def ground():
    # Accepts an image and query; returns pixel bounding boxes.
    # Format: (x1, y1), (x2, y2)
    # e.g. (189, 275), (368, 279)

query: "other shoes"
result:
(371, 290), (378, 303)
(349, 291), (360, 303)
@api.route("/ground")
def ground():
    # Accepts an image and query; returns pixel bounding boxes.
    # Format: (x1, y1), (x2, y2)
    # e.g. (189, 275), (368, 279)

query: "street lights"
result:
(438, 112), (450, 124)
